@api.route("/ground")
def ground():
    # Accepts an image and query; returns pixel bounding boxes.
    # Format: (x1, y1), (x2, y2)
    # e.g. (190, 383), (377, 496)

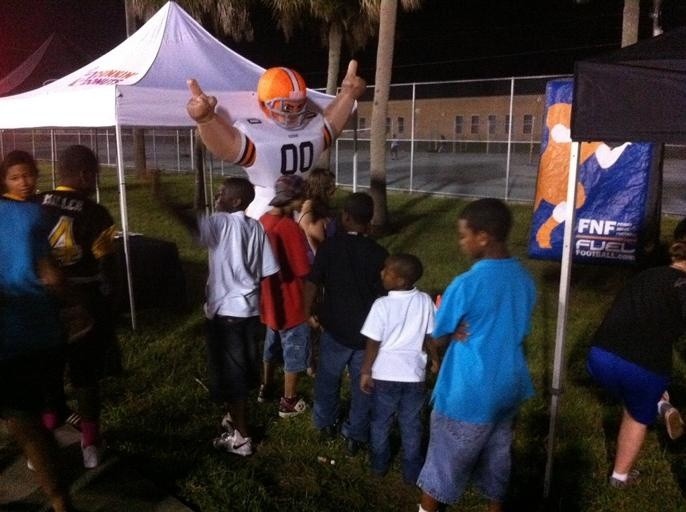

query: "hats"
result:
(269, 175), (305, 206)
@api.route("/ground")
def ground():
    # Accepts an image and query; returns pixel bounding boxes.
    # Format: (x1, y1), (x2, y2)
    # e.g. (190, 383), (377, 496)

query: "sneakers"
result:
(213, 412), (253, 456)
(344, 437), (359, 456)
(258, 384), (313, 418)
(320, 416), (340, 441)
(80, 433), (100, 468)
(660, 403), (684, 440)
(609, 470), (641, 489)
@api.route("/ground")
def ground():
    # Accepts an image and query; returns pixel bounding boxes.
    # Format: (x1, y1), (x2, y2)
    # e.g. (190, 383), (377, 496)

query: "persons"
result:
(415, 198), (536, 511)
(182, 59), (365, 216)
(146, 169), (287, 457)
(437, 133), (447, 155)
(388, 132), (399, 159)
(585, 218), (685, 489)
(260, 167), (390, 453)
(358, 252), (442, 484)
(0, 146), (115, 511)
